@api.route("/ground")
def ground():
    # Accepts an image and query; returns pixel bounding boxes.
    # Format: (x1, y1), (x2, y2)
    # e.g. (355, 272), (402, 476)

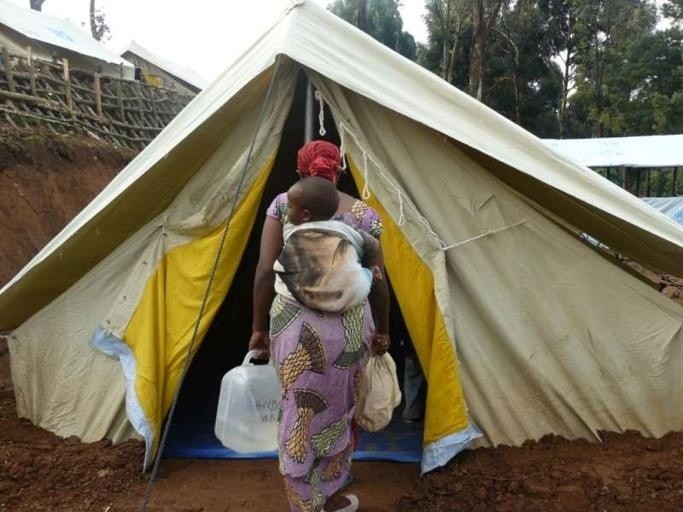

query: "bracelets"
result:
(374, 332), (390, 341)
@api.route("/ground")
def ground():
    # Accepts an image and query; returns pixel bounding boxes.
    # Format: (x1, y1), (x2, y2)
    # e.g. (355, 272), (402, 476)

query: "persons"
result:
(272, 174), (383, 315)
(248, 140), (391, 511)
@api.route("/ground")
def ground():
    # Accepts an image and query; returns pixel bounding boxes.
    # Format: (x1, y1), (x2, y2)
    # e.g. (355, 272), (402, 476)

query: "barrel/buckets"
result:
(215, 349), (283, 453)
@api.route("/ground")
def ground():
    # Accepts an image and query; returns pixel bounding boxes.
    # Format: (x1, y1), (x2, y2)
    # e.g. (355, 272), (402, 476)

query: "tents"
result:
(0, 0), (683, 477)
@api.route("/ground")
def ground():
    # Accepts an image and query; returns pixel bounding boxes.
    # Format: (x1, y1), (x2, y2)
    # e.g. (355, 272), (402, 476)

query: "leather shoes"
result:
(321, 495), (360, 512)
